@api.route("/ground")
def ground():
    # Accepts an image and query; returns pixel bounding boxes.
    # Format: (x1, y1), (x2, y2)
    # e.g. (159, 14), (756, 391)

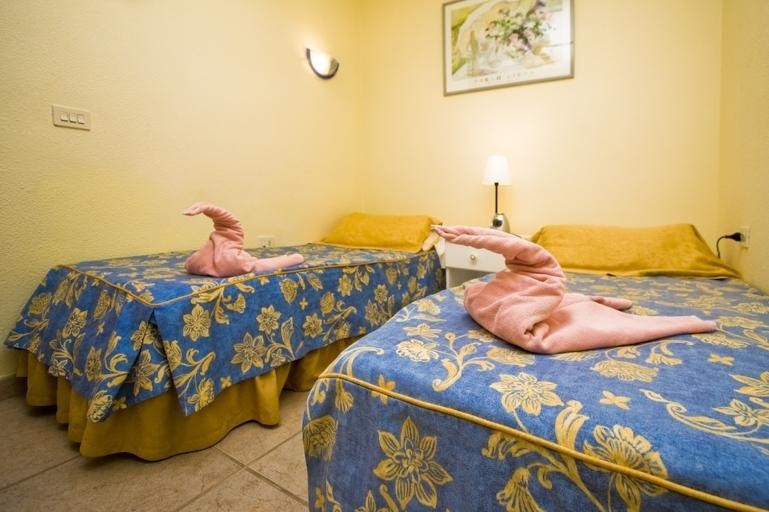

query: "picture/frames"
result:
(441, 0), (574, 95)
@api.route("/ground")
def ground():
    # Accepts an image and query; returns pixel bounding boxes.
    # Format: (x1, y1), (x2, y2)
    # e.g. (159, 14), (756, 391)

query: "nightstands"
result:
(443, 241), (508, 291)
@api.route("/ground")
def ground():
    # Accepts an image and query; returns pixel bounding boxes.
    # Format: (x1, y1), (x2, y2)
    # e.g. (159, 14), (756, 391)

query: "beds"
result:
(301, 223), (767, 512)
(6, 212), (446, 460)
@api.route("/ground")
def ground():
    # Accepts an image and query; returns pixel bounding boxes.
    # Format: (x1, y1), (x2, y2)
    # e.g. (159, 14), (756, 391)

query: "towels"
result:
(184, 202), (304, 278)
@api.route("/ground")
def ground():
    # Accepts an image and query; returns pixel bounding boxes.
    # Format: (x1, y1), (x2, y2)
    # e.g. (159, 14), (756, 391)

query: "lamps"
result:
(481, 154), (515, 234)
